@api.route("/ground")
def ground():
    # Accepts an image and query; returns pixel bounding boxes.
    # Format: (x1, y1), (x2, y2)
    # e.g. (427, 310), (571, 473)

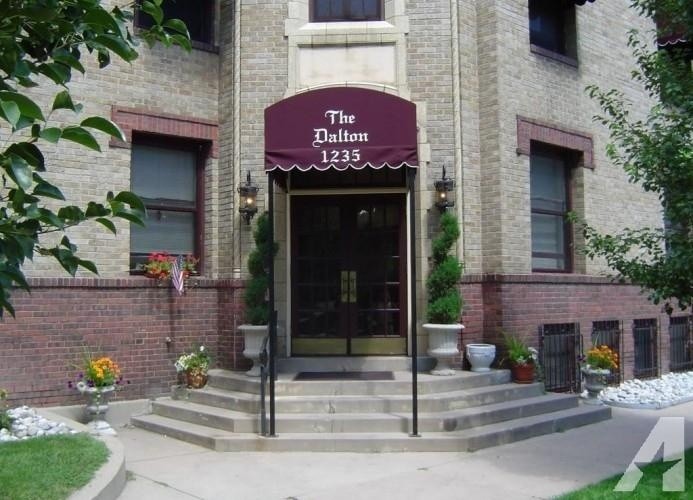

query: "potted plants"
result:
(237, 209), (280, 378)
(422, 207), (465, 377)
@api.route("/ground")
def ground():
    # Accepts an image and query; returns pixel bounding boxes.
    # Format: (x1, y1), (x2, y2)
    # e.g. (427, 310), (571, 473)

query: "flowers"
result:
(574, 330), (620, 372)
(142, 251), (201, 286)
(498, 327), (541, 369)
(175, 340), (212, 389)
(63, 337), (132, 433)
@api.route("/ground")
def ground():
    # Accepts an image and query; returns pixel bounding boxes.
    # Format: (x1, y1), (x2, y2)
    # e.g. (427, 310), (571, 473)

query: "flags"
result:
(171, 255), (185, 296)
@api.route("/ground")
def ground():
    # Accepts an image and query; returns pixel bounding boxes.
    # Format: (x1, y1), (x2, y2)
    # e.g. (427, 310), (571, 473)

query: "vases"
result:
(466, 340), (497, 373)
(512, 363), (535, 381)
(579, 366), (611, 405)
(76, 386), (118, 436)
(185, 369), (208, 389)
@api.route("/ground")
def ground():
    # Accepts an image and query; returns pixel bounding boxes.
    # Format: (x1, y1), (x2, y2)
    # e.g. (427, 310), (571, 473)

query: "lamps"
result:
(434, 163), (456, 215)
(236, 171), (260, 226)
